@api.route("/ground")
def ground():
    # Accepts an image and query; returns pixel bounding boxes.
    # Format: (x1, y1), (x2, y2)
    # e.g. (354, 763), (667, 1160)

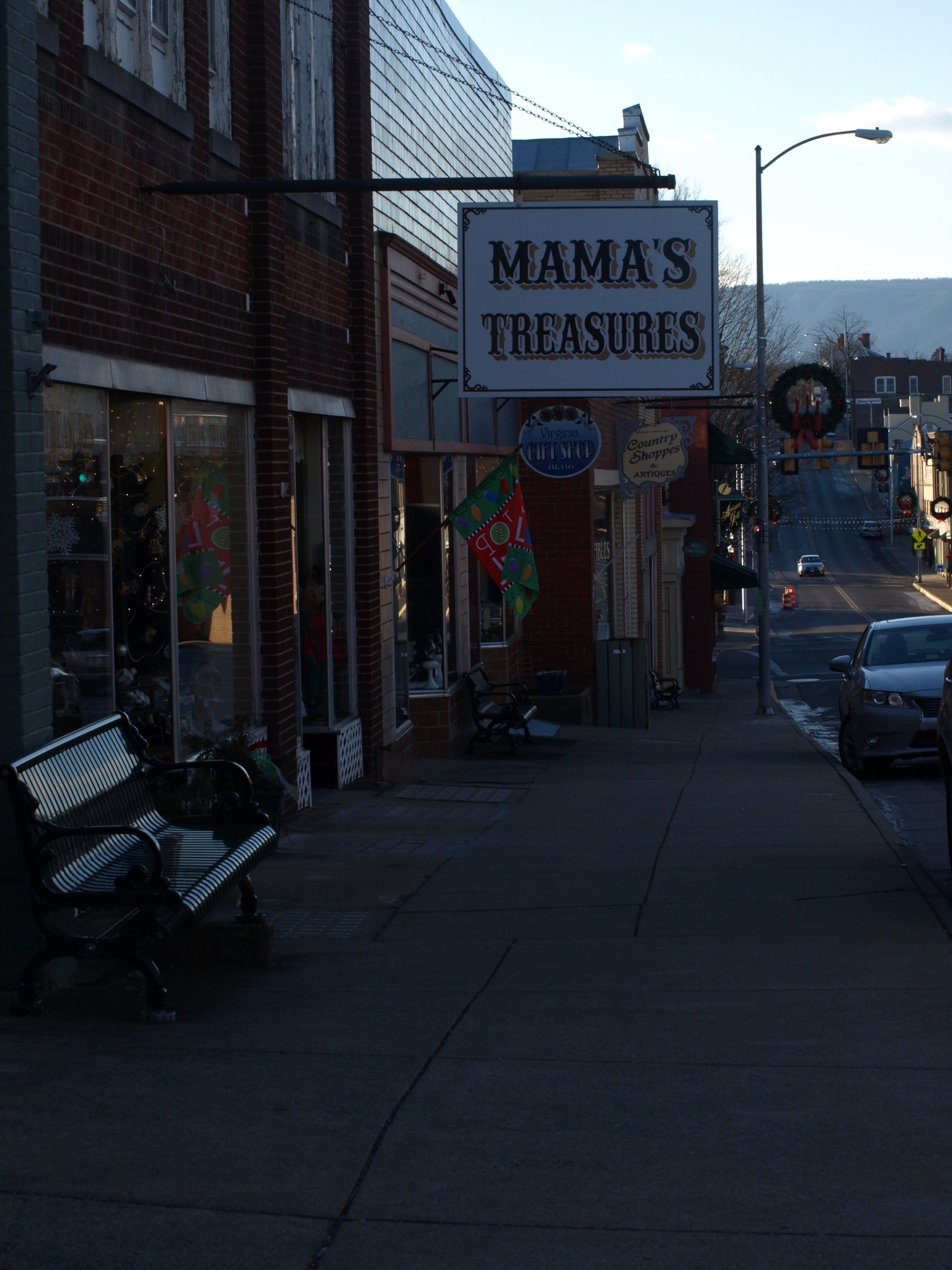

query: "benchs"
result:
(463, 667), (537, 756)
(2, 708), (282, 1024)
(648, 669), (682, 710)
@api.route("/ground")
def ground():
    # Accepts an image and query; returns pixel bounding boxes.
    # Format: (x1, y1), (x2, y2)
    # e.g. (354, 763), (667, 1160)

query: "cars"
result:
(829, 614), (951, 778)
(797, 555), (826, 576)
(859, 520), (882, 538)
(938, 661), (952, 854)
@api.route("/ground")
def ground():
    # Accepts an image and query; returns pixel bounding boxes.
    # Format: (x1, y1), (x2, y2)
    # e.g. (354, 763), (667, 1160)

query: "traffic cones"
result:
(783, 586), (796, 609)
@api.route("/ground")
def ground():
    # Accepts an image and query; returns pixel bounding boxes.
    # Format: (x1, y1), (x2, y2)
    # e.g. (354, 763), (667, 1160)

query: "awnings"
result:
(713, 552), (758, 593)
(709, 420), (756, 464)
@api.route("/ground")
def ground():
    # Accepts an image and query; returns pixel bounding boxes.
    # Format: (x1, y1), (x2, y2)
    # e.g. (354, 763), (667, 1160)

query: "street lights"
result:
(845, 397), (874, 504)
(753, 127), (897, 710)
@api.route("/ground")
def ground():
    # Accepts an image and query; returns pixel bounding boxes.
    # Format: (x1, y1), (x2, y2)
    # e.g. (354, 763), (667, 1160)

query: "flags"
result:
(450, 453), (539, 622)
